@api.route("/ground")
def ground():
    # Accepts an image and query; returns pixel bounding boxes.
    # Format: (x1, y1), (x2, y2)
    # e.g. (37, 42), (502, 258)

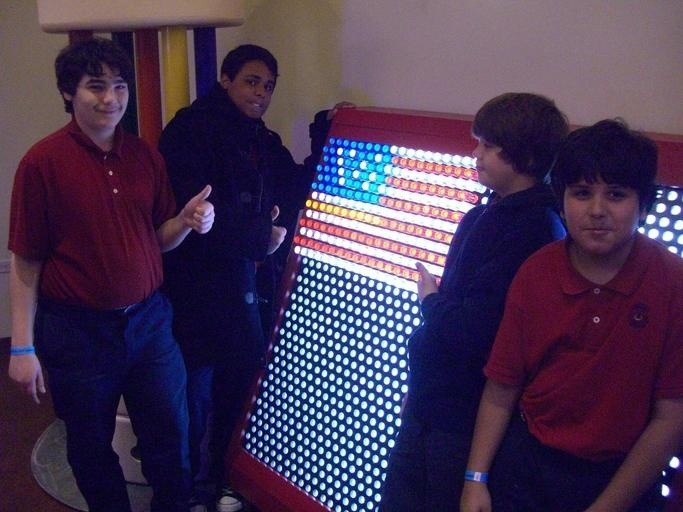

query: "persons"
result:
(455, 118), (682, 511)
(156, 43), (356, 512)
(375, 95), (570, 511)
(6, 33), (214, 512)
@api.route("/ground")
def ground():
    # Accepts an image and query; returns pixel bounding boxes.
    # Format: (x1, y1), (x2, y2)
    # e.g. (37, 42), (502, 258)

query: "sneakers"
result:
(188, 484), (242, 512)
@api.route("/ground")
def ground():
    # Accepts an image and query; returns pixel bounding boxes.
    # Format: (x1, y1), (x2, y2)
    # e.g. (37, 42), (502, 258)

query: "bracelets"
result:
(463, 471), (491, 483)
(7, 345), (36, 358)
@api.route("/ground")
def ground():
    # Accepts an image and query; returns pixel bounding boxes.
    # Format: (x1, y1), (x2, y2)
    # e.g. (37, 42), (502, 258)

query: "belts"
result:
(40, 298), (141, 320)
